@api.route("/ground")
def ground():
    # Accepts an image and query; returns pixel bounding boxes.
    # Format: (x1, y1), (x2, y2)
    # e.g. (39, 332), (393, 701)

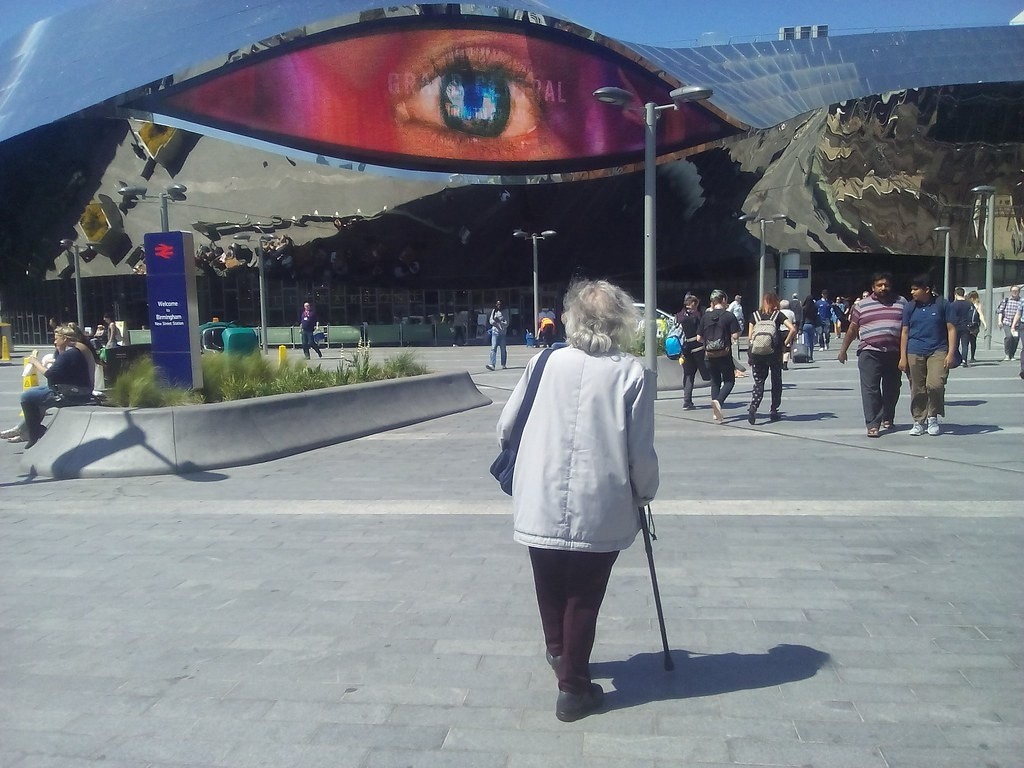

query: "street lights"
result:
(513, 229), (557, 336)
(739, 210), (797, 312)
(233, 234), (278, 353)
(593, 82), (715, 399)
(117, 183), (186, 234)
(970, 184), (995, 352)
(933, 226), (962, 308)
(60, 240), (102, 334)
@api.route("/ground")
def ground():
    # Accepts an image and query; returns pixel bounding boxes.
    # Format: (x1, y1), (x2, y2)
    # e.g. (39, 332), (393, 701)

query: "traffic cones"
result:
(1, 336), (11, 361)
(19, 357), (53, 416)
(278, 345), (288, 370)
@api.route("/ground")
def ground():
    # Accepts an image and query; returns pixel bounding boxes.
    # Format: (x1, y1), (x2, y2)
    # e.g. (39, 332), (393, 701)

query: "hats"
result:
(821, 289), (828, 293)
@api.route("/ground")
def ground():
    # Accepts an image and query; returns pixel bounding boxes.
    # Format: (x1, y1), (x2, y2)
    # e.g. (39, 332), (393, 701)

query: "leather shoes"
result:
(545, 648), (565, 678)
(556, 683), (604, 722)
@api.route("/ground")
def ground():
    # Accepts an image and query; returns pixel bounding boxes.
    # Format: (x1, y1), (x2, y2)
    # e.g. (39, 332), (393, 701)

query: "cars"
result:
(632, 303), (676, 330)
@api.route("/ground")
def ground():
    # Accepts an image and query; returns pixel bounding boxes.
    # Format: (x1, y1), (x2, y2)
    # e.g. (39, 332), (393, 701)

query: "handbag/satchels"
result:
(114, 326), (122, 342)
(489, 444), (516, 496)
(49, 382), (91, 408)
(815, 315), (822, 328)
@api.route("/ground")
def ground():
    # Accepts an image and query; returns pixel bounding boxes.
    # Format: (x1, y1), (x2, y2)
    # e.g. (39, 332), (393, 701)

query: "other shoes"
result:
(971, 359), (975, 362)
(679, 386), (894, 437)
(319, 353), (322, 358)
(783, 338), (832, 370)
(960, 359), (968, 367)
(305, 356), (310, 360)
(0, 423), (47, 450)
(501, 365), (506, 369)
(486, 364), (495, 371)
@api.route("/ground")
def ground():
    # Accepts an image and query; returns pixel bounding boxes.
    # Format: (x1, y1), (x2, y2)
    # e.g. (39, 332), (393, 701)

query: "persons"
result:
(524, 306), (556, 348)
(496, 279), (661, 721)
(451, 306), (467, 347)
(299, 302), (322, 360)
(486, 299), (510, 372)
(639, 271), (1024, 437)
(0, 312), (117, 450)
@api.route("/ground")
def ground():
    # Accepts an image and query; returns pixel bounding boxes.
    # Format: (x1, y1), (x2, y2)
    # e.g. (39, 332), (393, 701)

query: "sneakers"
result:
(909, 421), (925, 436)
(926, 417), (940, 435)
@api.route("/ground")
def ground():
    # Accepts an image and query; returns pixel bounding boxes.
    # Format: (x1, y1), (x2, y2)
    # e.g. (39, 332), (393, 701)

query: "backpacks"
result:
(752, 311), (778, 356)
(965, 306), (981, 332)
(702, 310), (727, 352)
(664, 321), (688, 365)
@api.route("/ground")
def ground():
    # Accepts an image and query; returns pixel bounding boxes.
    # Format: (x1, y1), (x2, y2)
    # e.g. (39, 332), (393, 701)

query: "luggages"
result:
(791, 332), (808, 363)
